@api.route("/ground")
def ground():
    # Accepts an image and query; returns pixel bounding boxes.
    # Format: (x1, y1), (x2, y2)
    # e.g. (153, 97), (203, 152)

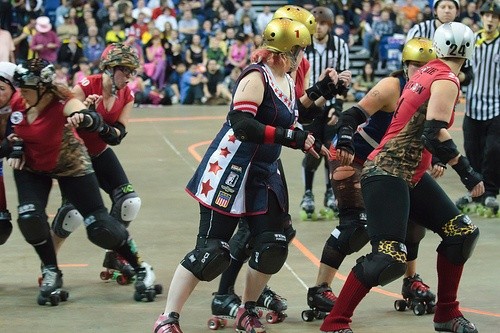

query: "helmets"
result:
(273, 5), (317, 34)
(310, 7), (335, 25)
(480, 0), (500, 16)
(260, 19), (312, 52)
(15, 58), (56, 90)
(0, 62), (19, 90)
(97, 43), (141, 70)
(433, 0), (460, 9)
(433, 22), (475, 59)
(401, 38), (436, 62)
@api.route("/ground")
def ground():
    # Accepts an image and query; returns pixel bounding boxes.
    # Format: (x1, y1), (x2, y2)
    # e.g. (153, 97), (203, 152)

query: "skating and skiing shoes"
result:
(301, 191), (318, 220)
(477, 193), (500, 218)
(394, 273), (436, 316)
(319, 189), (340, 218)
(37, 266), (69, 306)
(456, 195), (477, 213)
(133, 262), (163, 302)
(100, 250), (136, 285)
(256, 285), (288, 324)
(302, 282), (338, 322)
(208, 292), (242, 329)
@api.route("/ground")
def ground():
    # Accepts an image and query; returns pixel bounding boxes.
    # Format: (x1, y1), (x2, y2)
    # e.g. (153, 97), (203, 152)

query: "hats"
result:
(36, 15), (52, 32)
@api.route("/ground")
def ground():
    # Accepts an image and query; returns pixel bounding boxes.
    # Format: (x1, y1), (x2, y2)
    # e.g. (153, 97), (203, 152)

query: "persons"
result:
(0, 0), (500, 333)
(319, 23), (486, 333)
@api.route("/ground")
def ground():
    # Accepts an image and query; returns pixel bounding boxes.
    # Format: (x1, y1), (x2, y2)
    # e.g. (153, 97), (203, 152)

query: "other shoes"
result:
(233, 305), (266, 333)
(153, 312), (183, 333)
(434, 316), (477, 333)
(320, 328), (353, 333)
